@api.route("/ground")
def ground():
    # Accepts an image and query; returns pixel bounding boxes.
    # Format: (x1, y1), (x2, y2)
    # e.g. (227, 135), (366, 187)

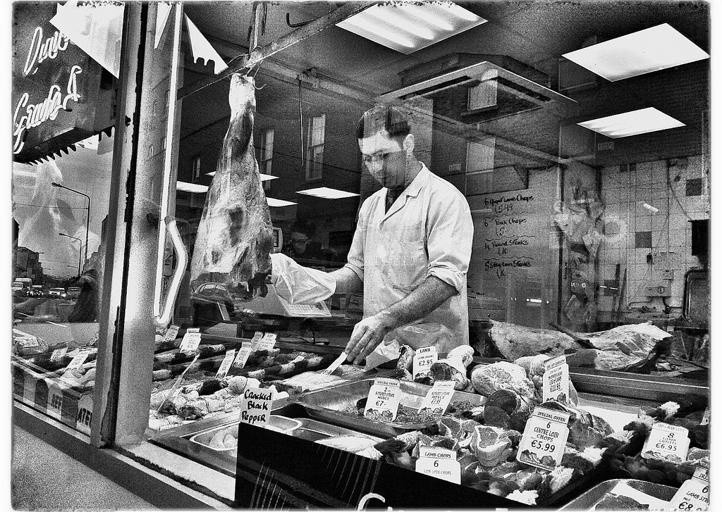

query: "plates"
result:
(188, 414), (303, 454)
(295, 376), (490, 439)
(147, 381), (291, 438)
(552, 476), (678, 512)
(539, 349), (710, 404)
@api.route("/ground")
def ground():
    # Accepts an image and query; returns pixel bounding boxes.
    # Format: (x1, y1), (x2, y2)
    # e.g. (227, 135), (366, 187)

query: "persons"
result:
(270, 101), (474, 370)
